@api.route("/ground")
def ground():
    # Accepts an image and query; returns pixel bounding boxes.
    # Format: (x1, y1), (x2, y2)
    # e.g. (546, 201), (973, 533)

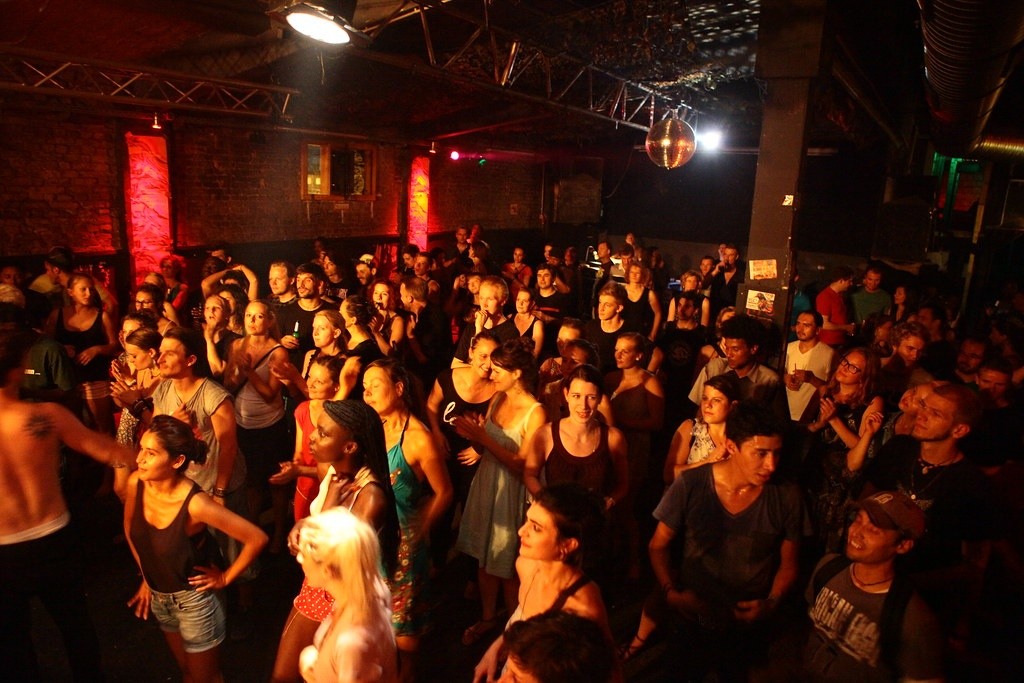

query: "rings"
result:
(118, 370), (120, 373)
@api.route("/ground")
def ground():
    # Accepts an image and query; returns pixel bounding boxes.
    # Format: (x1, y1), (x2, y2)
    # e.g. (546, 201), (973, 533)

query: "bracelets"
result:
(213, 488), (226, 496)
(128, 379), (136, 387)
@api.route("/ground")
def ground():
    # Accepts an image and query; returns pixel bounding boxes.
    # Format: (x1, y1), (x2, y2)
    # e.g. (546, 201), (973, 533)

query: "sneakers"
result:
(231, 613), (251, 639)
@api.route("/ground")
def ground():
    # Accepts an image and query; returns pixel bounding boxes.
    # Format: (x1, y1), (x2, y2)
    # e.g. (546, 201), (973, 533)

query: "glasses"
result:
(841, 358), (866, 376)
(917, 400), (956, 424)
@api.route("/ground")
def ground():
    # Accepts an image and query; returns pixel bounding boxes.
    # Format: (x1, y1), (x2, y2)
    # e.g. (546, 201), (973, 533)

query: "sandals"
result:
(616, 640), (639, 661)
(463, 617), (498, 648)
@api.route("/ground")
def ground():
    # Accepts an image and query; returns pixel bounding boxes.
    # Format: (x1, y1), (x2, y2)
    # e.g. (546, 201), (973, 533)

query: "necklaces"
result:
(579, 424), (596, 453)
(520, 570), (574, 622)
(149, 369), (161, 378)
(852, 563), (892, 586)
(920, 455), (954, 474)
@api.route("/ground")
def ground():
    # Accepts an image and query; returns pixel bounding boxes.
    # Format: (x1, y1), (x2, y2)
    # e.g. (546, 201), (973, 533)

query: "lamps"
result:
(279, 0), (359, 44)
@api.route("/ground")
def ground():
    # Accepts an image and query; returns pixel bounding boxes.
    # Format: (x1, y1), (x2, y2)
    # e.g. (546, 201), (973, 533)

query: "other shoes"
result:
(95, 485), (113, 498)
(269, 536), (287, 553)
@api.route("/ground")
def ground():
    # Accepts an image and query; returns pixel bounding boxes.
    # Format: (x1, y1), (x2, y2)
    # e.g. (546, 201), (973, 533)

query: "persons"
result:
(0, 222), (1024, 683)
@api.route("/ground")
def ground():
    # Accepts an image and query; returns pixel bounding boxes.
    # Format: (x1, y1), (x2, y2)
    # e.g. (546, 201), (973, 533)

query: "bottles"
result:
(292, 320), (300, 338)
(719, 260), (729, 272)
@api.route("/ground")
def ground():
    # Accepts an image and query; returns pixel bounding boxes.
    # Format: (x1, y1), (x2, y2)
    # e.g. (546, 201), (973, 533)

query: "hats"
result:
(850, 491), (924, 536)
(351, 254), (380, 269)
